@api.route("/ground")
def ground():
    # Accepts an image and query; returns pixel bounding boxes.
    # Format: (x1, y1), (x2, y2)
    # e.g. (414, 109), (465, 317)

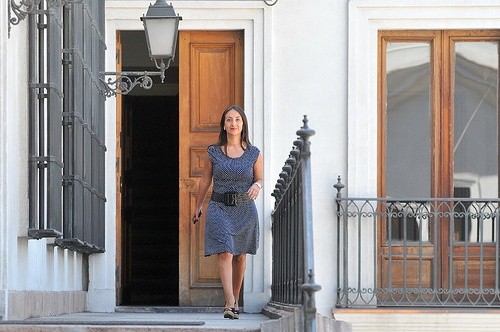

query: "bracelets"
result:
(254, 182), (262, 189)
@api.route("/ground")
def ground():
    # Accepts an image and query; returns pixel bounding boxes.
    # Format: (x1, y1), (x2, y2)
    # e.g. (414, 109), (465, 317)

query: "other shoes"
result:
(224, 308), (235, 319)
(234, 310), (239, 319)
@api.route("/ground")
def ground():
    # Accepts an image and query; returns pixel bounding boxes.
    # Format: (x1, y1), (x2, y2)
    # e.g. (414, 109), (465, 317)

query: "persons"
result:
(192, 106), (264, 320)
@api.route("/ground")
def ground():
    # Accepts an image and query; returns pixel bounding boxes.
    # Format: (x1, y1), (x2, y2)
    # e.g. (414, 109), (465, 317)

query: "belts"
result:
(211, 190), (249, 206)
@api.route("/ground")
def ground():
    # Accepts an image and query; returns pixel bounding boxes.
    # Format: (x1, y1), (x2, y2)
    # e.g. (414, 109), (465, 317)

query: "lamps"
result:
(101, 0), (183, 98)
